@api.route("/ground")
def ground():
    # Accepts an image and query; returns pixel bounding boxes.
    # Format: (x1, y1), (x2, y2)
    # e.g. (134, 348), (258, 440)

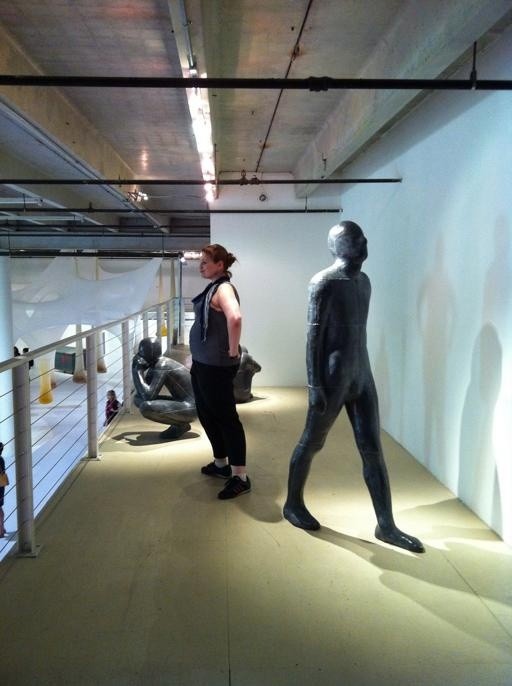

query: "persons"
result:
(232, 344), (262, 403)
(14, 346), (21, 358)
(131, 336), (199, 440)
(23, 347), (35, 369)
(0, 442), (7, 538)
(102, 390), (123, 426)
(282, 218), (424, 555)
(188, 245), (256, 501)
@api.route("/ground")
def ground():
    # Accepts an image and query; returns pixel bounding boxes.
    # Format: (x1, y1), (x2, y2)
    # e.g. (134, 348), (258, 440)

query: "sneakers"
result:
(216, 475), (251, 499)
(200, 460), (231, 478)
